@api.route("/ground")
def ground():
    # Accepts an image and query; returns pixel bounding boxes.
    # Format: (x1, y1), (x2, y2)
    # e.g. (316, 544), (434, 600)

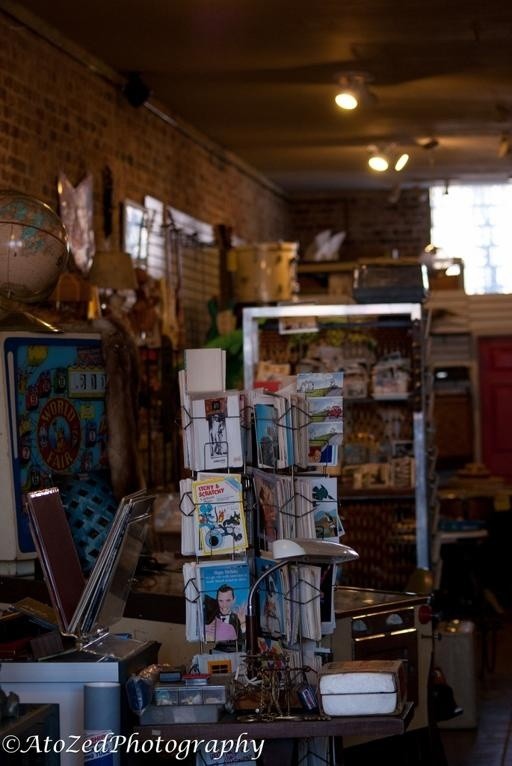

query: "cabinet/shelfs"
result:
(243, 297), (431, 577)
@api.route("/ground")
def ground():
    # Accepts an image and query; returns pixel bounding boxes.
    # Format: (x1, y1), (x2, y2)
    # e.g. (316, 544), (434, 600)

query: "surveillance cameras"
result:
(115, 67), (148, 109)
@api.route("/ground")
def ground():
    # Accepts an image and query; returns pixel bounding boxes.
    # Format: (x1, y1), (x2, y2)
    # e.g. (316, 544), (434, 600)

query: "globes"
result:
(0, 190), (69, 333)
